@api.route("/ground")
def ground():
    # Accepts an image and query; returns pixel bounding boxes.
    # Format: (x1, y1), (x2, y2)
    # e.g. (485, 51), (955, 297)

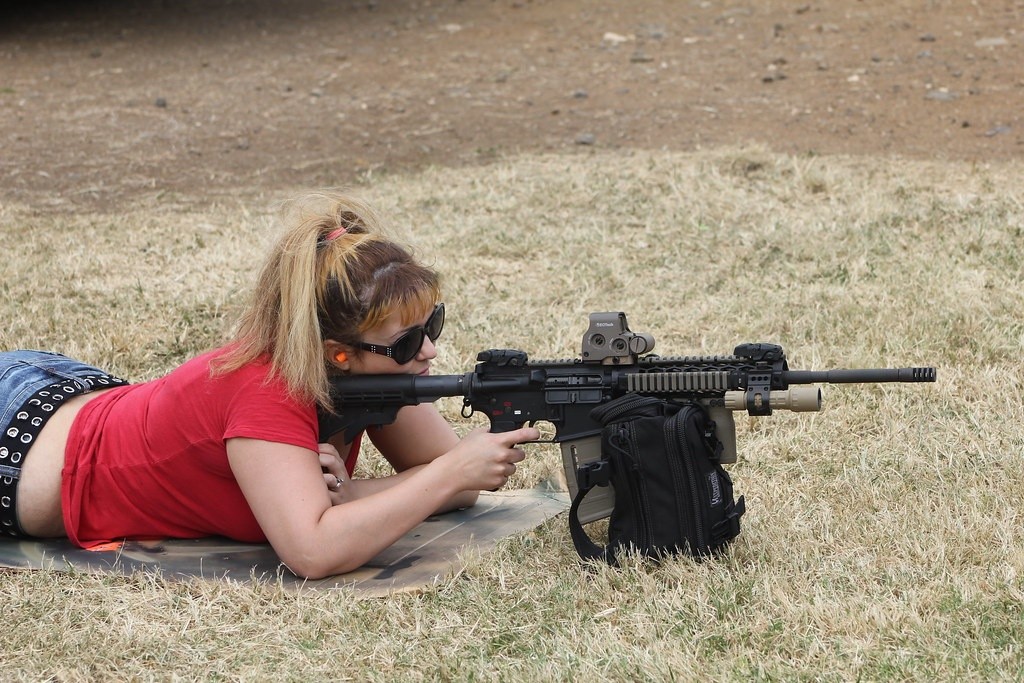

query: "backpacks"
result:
(569, 395), (747, 563)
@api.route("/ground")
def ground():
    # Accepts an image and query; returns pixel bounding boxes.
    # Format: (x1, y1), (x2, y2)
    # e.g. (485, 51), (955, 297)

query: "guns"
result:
(314, 311), (938, 526)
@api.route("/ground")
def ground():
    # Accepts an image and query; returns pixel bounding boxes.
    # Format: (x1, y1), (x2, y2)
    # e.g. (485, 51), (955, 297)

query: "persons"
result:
(0, 190), (540, 582)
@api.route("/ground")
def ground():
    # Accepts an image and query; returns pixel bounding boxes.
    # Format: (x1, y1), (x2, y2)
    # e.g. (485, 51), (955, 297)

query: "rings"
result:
(329, 477), (344, 491)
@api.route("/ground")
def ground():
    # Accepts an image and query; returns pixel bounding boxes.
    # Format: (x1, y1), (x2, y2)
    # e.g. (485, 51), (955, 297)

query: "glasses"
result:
(335, 304), (445, 365)
